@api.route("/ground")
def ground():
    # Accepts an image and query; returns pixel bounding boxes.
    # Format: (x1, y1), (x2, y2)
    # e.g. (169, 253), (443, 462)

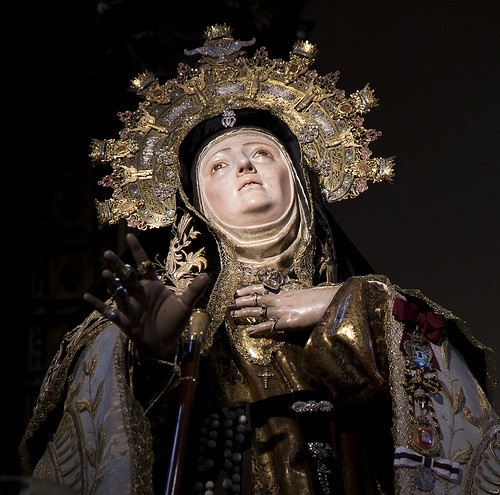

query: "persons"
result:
(18, 21), (500, 495)
